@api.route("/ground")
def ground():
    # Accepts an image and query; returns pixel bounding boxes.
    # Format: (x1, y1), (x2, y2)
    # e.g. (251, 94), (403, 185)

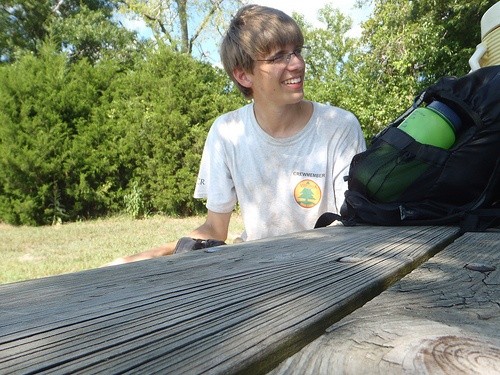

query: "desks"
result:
(0, 221), (500, 372)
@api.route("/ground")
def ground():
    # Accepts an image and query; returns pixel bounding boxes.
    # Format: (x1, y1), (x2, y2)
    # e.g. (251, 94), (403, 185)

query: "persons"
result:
(106, 4), (368, 268)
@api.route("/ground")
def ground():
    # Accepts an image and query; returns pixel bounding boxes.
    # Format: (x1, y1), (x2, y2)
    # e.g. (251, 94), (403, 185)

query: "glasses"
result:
(250, 46), (311, 64)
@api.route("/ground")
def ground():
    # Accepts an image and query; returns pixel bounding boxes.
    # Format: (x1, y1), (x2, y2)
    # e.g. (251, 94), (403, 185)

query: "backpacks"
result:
(314, 64), (500, 232)
